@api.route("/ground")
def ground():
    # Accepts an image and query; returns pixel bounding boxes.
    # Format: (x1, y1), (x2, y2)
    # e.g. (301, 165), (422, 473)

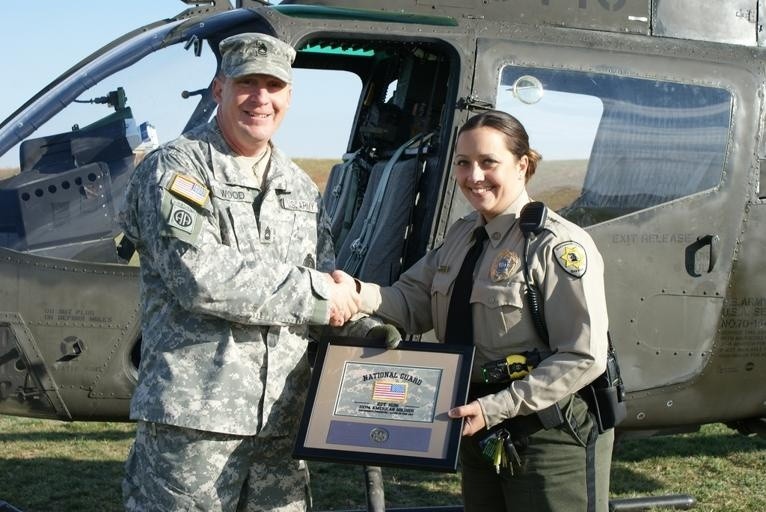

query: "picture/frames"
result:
(291, 334), (476, 473)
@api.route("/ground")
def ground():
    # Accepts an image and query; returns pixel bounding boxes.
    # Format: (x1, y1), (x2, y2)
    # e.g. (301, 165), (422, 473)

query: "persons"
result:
(332, 111), (627, 511)
(122, 31), (405, 510)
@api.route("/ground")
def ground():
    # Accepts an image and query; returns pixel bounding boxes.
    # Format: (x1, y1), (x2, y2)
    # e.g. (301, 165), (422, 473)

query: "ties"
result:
(445, 226), (489, 345)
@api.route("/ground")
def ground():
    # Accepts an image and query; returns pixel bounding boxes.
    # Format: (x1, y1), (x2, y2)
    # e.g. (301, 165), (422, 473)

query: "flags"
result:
(371, 380), (409, 402)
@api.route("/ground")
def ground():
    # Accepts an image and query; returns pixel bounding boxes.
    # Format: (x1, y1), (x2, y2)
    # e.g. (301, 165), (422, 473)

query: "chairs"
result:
(323, 163), (368, 258)
(334, 157), (422, 287)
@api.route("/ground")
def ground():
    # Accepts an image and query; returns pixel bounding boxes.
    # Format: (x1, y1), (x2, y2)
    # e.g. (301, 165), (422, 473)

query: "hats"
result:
(219, 32), (296, 83)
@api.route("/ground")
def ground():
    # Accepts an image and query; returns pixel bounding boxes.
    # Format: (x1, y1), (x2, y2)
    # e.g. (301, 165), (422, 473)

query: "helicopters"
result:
(0, 0), (765, 512)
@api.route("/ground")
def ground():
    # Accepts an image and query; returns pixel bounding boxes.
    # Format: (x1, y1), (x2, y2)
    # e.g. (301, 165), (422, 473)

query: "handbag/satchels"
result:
(582, 386), (627, 433)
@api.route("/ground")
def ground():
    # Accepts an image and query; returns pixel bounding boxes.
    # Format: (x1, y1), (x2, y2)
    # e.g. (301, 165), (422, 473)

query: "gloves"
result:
(366, 324), (402, 349)
